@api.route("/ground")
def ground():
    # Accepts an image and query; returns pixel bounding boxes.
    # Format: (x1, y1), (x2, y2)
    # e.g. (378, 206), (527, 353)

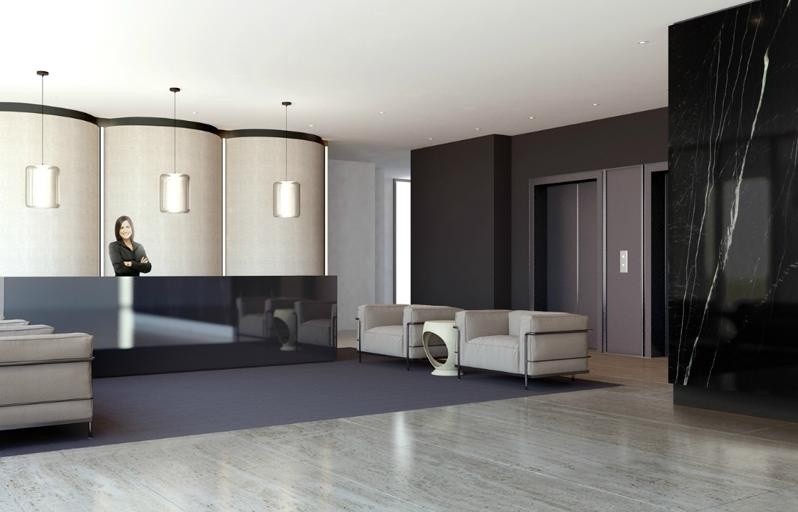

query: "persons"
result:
(108, 215), (152, 276)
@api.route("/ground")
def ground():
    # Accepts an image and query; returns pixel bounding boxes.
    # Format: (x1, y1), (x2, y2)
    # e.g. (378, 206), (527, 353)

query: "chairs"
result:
(455, 308), (591, 389)
(236, 294), (300, 337)
(295, 299), (336, 347)
(355, 303), (465, 371)
(1, 319), (54, 329)
(0, 328), (94, 435)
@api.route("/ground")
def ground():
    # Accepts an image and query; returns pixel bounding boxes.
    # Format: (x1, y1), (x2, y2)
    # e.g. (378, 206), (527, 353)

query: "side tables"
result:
(422, 319), (464, 378)
(273, 309), (301, 351)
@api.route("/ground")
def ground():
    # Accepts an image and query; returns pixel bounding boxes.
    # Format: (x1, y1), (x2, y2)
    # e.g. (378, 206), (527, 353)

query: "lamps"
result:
(273, 101), (301, 219)
(160, 87), (190, 214)
(26, 71), (59, 209)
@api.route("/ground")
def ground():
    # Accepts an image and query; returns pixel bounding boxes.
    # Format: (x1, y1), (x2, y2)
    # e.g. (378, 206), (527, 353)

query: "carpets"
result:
(0, 349), (624, 459)
